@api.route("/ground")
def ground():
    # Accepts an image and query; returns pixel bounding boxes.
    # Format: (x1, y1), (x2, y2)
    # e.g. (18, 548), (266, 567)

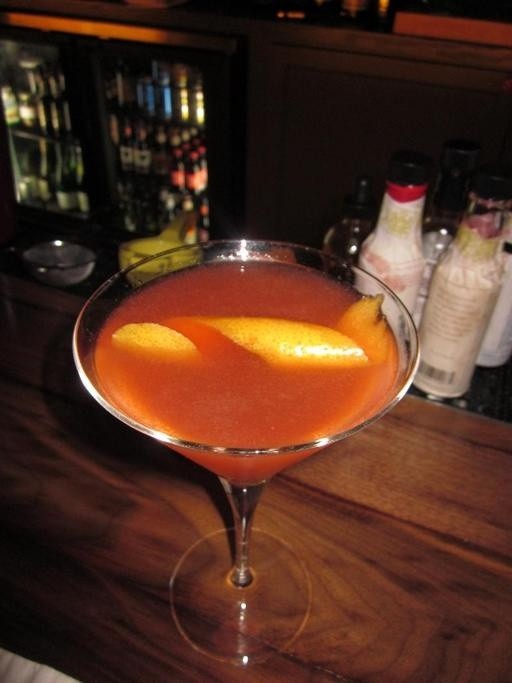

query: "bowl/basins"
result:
(21, 237), (97, 286)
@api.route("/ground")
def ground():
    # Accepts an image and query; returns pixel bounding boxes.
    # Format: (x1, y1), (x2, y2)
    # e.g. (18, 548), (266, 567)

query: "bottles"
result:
(335, 201), (366, 277)
(425, 224), (446, 311)
(425, 218), (502, 399)
(1, 52), (213, 240)
(475, 244), (510, 367)
(366, 180), (422, 344)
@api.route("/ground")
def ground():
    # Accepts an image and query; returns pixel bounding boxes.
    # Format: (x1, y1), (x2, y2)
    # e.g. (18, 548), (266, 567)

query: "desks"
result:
(1, 271), (512, 683)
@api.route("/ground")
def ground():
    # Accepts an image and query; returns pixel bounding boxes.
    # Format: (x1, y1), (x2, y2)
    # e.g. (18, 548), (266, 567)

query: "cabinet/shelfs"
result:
(0, 37), (251, 255)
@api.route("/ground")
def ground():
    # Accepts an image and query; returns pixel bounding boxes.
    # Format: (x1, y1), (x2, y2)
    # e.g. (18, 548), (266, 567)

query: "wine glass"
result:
(69, 242), (420, 667)
